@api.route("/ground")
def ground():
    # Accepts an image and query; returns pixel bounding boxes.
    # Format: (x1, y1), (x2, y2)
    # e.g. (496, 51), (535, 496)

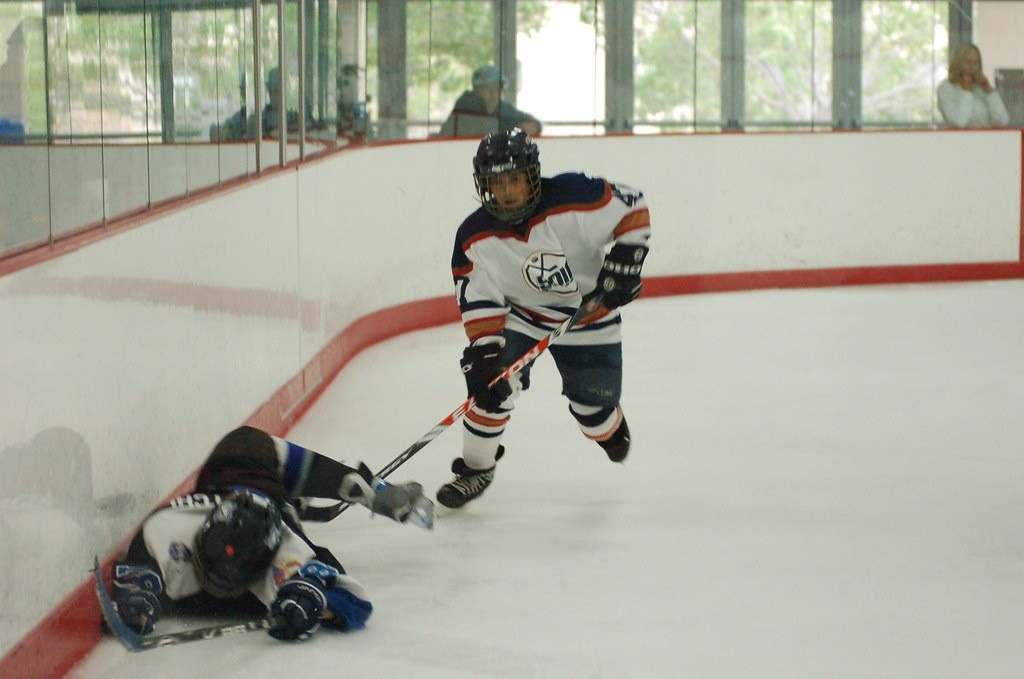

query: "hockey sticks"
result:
(298, 295), (602, 524)
(92, 555), (285, 651)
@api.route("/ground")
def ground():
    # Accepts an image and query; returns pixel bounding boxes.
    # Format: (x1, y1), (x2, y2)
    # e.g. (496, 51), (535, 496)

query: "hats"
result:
(472, 66), (506, 86)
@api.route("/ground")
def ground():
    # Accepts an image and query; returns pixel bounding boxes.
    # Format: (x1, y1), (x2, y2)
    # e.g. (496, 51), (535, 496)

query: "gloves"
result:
(596, 244), (649, 310)
(459, 343), (520, 413)
(115, 591), (160, 635)
(266, 581), (325, 642)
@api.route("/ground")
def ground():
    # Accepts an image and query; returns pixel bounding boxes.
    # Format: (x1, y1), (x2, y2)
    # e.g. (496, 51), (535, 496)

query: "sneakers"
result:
(598, 414), (631, 463)
(436, 444), (507, 509)
(371, 482), (434, 529)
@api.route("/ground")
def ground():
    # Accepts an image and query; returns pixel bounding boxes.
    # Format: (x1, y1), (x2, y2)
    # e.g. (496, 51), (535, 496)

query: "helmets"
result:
(192, 490), (282, 598)
(473, 128), (540, 194)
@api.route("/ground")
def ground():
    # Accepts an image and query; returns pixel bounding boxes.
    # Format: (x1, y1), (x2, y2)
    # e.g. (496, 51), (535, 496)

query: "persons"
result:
(935, 43), (1010, 127)
(435, 127), (652, 509)
(97, 425), (434, 642)
(442, 65), (543, 137)
(210, 64), (375, 140)
